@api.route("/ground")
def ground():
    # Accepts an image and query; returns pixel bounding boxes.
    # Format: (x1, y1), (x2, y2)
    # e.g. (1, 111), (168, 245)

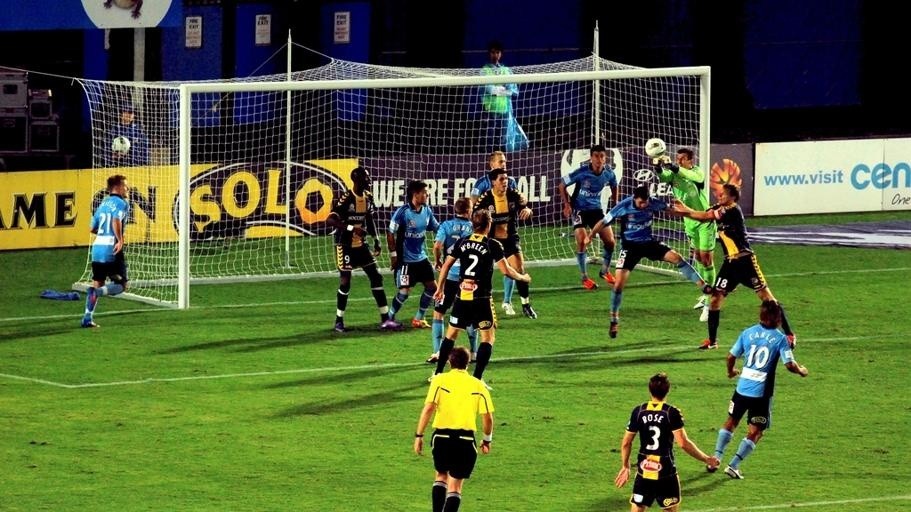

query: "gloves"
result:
(653, 154), (678, 174)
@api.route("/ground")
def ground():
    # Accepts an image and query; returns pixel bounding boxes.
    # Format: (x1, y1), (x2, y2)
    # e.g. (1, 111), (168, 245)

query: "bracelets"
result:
(483, 432), (492, 441)
(415, 433), (424, 437)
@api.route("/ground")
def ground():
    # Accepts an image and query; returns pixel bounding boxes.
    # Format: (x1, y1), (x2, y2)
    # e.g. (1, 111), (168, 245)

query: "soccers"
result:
(645, 138), (665, 158)
(711, 159), (742, 199)
(112, 137), (130, 155)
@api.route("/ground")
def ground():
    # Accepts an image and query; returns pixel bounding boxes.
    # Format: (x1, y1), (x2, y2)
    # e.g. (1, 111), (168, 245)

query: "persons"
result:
(414, 344), (493, 512)
(707, 300), (810, 478)
(653, 149), (801, 352)
(615, 373), (720, 512)
(325, 166), (399, 335)
(426, 152), (537, 389)
(108, 105), (149, 169)
(98, 102), (145, 168)
(558, 147), (712, 338)
(81, 174), (133, 329)
(481, 46), (522, 156)
(387, 180), (441, 329)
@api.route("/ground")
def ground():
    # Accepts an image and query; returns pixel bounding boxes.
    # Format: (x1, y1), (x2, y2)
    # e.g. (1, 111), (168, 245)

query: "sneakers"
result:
(609, 312), (619, 339)
(468, 352), (478, 363)
(522, 304), (537, 319)
(693, 281), (713, 323)
(722, 462), (745, 481)
(581, 269), (616, 291)
(786, 332), (797, 352)
(425, 350), (441, 362)
(334, 321), (348, 333)
(705, 460), (721, 473)
(481, 378), (493, 391)
(377, 315), (433, 331)
(81, 321), (101, 328)
(427, 371), (442, 382)
(697, 337), (719, 352)
(87, 287), (97, 312)
(501, 301), (516, 315)
